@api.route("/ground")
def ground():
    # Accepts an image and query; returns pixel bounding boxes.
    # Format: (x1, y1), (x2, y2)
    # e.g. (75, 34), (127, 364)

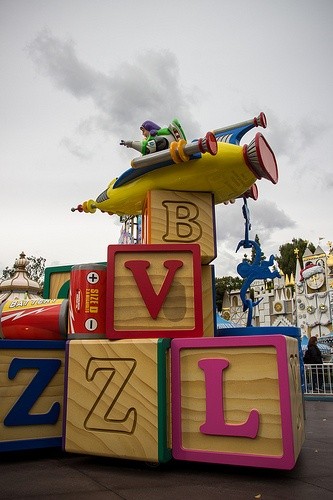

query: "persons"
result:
(121, 120), (176, 154)
(303, 336), (325, 387)
